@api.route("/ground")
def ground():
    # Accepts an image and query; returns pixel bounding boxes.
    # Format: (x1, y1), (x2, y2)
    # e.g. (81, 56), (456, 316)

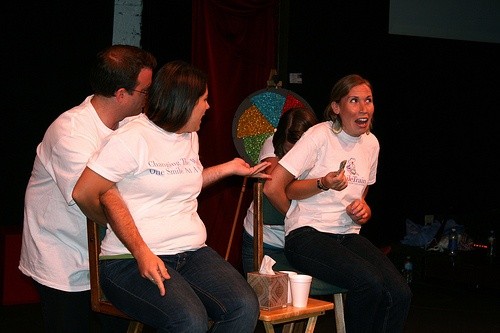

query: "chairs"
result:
(87, 182), (350, 333)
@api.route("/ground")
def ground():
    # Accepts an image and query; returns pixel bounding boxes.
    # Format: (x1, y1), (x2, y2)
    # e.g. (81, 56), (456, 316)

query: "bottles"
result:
(449, 229), (458, 256)
(487, 231), (496, 255)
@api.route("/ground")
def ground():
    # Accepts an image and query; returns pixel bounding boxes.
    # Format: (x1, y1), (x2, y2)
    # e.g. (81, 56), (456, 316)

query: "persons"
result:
(72, 62), (261, 332)
(18, 44), (272, 333)
(262, 74), (415, 333)
(241, 106), (370, 280)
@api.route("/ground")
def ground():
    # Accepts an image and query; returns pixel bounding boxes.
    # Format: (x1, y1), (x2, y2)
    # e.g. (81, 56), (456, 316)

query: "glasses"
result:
(126, 86), (151, 97)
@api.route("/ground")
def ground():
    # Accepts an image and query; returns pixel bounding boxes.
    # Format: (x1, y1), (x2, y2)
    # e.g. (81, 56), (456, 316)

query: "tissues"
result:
(246, 255), (288, 311)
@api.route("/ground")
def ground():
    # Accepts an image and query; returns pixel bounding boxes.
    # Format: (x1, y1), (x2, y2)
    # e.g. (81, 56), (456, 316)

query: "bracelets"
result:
(321, 176), (328, 189)
(317, 178), (325, 191)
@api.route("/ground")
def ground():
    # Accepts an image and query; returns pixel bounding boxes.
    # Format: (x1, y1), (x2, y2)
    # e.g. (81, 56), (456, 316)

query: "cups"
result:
(290, 275), (313, 307)
(279, 271), (298, 303)
